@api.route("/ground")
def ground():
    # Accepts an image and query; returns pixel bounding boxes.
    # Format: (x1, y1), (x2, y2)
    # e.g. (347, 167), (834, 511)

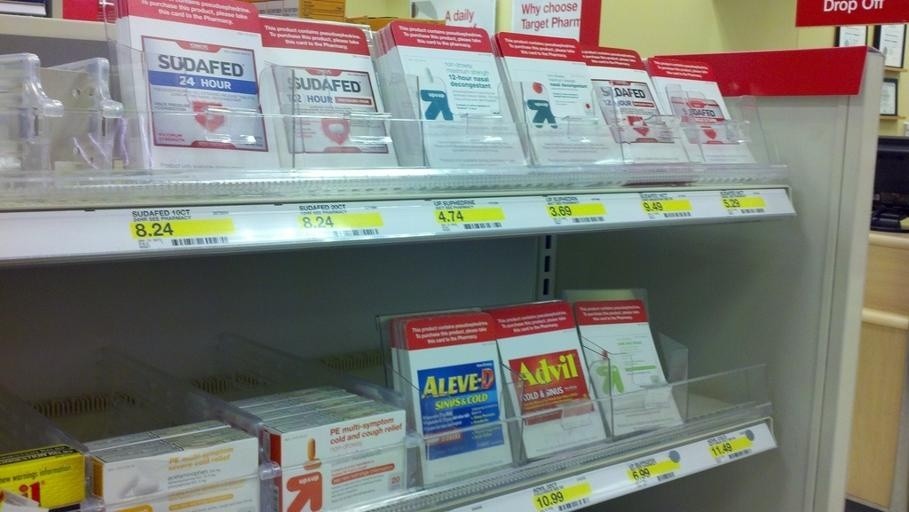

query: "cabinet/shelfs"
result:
(0, 14), (889, 511)
